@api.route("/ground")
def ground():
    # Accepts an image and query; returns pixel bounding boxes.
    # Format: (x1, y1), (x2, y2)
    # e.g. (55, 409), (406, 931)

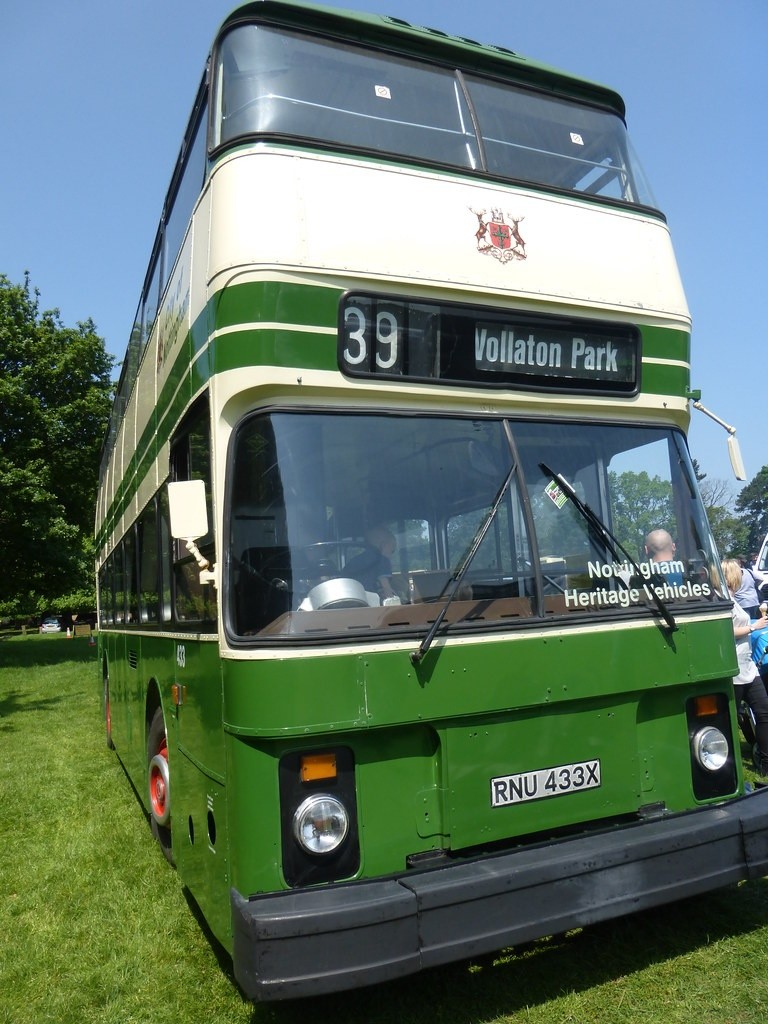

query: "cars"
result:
(41, 618), (63, 632)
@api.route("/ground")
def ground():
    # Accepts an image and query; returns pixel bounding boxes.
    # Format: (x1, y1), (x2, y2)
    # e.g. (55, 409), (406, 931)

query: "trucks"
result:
(752, 533), (768, 610)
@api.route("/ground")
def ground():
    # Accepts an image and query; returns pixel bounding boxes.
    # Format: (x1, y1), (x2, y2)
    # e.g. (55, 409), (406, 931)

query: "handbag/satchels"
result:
(748, 569), (768, 602)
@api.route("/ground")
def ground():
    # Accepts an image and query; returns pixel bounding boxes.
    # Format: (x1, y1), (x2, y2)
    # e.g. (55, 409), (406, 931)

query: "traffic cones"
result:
(88, 633), (95, 646)
(66, 627), (70, 638)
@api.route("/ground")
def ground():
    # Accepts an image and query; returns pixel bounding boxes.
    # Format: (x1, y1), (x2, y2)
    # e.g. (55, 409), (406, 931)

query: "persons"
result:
(343, 530), (397, 604)
(644, 529), (682, 590)
(710, 551), (768, 777)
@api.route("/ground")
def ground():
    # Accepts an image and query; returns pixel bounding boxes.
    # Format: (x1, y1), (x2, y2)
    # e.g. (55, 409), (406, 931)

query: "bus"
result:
(94, 0), (768, 1003)
